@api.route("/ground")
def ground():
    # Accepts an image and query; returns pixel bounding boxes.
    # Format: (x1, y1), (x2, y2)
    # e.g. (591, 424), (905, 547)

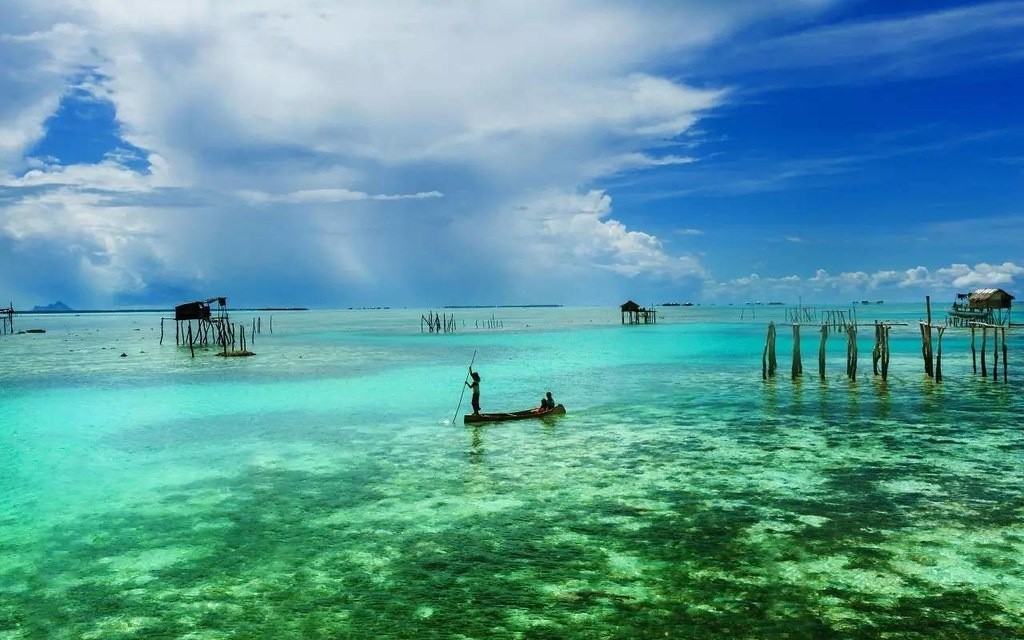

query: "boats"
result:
(463, 404), (569, 425)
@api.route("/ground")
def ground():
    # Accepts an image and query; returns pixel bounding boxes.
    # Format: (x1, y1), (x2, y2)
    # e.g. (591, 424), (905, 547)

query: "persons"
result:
(538, 391), (555, 413)
(465, 366), (481, 415)
